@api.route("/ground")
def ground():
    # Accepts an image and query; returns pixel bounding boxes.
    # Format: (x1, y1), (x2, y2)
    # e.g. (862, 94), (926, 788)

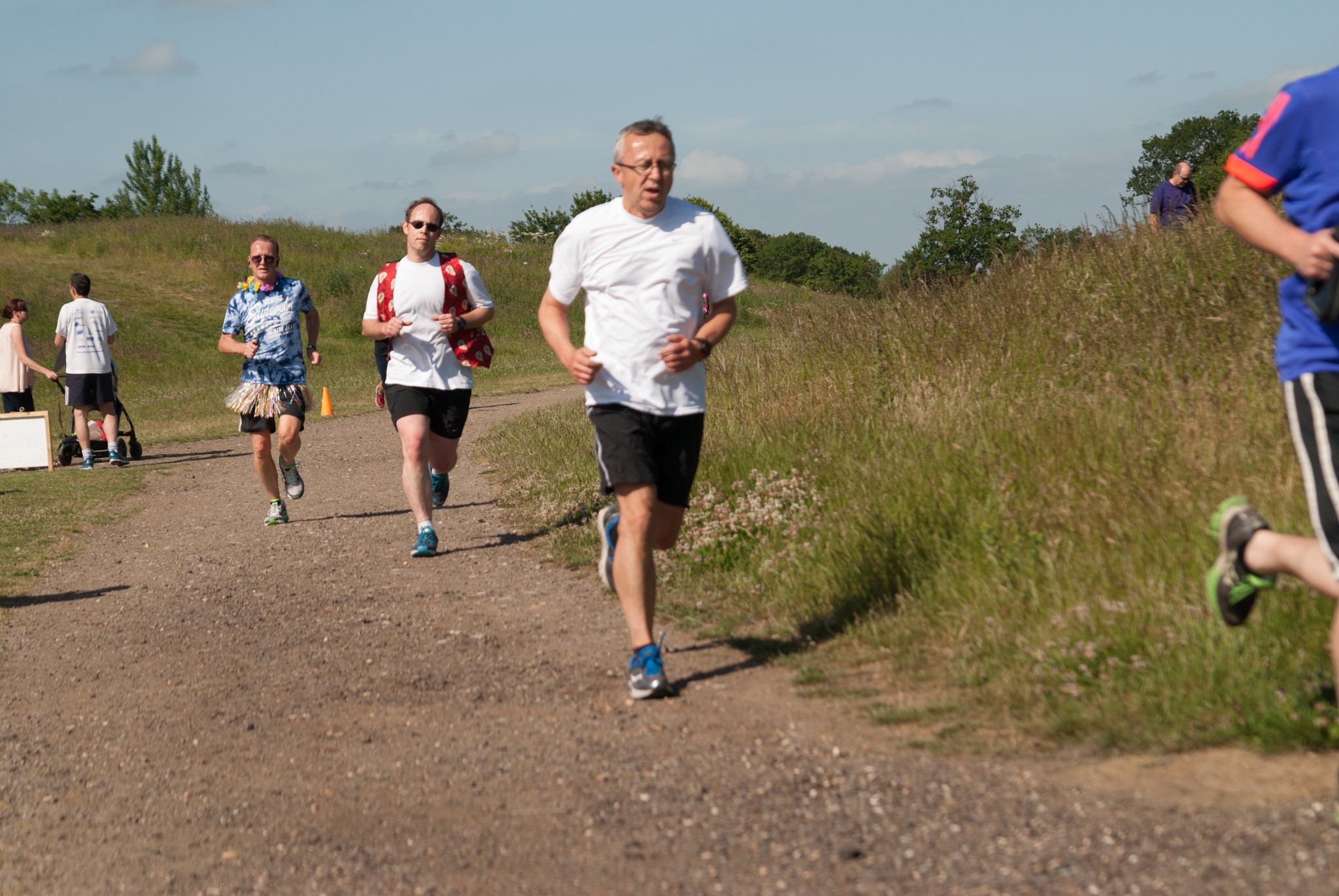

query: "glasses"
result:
(252, 254), (275, 264)
(17, 307), (27, 311)
(1177, 175), (1190, 181)
(616, 163), (678, 176)
(407, 221), (439, 232)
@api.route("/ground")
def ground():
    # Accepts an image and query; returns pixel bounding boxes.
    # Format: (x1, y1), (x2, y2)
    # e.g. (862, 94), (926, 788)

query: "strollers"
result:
(53, 373), (142, 466)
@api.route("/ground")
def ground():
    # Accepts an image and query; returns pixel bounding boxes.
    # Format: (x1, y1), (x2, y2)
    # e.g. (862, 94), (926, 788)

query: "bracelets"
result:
(306, 344), (317, 350)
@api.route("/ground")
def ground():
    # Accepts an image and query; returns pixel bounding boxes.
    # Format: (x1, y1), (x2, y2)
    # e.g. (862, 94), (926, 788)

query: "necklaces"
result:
(237, 269), (284, 293)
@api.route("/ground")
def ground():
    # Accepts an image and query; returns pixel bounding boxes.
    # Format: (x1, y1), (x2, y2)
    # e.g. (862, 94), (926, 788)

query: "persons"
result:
(361, 198), (496, 557)
(0, 298), (57, 470)
(1150, 161), (1198, 234)
(536, 120), (749, 699)
(54, 272), (130, 469)
(218, 235), (323, 526)
(1204, 64), (1339, 709)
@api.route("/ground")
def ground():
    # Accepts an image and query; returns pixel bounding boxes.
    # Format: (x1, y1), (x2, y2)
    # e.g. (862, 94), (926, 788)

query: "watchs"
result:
(693, 337), (711, 360)
(456, 315), (467, 330)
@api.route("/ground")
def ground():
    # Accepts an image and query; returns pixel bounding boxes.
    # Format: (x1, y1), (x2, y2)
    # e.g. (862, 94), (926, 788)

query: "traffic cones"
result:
(321, 387), (334, 417)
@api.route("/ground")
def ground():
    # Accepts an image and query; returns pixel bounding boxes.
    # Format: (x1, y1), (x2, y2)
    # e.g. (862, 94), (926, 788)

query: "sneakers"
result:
(80, 457), (94, 470)
(1206, 495), (1278, 627)
(411, 527), (438, 557)
(109, 450), (130, 465)
(429, 465), (449, 506)
(278, 454), (304, 500)
(597, 506), (621, 594)
(264, 499), (289, 526)
(627, 633), (666, 699)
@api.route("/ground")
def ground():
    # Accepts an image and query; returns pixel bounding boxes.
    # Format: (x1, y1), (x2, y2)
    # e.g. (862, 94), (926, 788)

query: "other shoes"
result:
(53, 460), (62, 467)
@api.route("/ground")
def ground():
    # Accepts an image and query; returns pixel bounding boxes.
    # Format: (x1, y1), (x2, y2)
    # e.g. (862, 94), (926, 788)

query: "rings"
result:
(443, 324), (446, 329)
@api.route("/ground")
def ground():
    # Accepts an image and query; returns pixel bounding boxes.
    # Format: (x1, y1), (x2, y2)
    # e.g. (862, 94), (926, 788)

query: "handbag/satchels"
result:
(87, 419), (107, 441)
